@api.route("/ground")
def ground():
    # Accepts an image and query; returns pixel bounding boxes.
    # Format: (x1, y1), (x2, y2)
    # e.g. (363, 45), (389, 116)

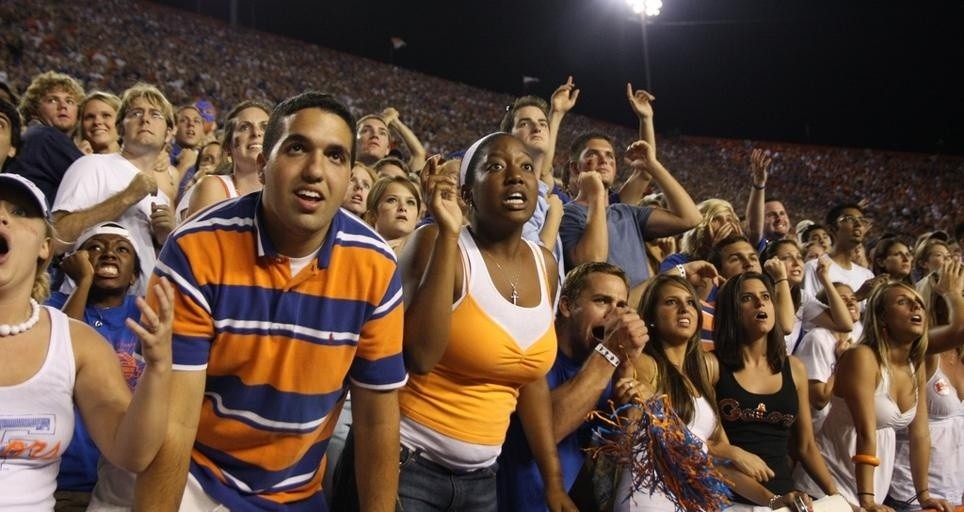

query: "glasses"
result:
(838, 215), (870, 227)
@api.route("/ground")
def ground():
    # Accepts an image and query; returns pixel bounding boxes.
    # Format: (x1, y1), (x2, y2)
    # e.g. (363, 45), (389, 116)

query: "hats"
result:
(74, 220), (143, 270)
(914, 229), (949, 247)
(0, 173), (50, 223)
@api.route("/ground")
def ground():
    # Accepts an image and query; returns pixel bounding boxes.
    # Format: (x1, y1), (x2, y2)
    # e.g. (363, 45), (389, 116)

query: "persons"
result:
(1, 0), (964, 511)
